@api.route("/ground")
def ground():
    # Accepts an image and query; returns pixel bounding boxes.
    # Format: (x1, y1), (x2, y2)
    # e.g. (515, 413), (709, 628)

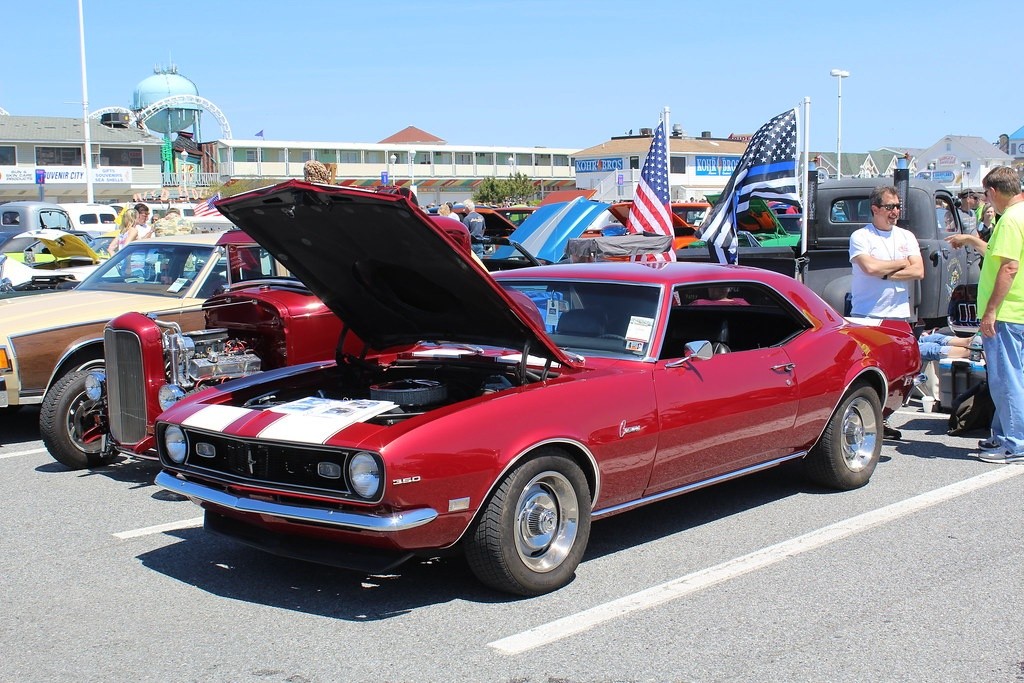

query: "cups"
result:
(922, 396), (934, 413)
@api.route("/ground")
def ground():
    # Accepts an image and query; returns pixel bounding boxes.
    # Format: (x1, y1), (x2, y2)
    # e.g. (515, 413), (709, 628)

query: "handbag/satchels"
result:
(949, 379), (996, 436)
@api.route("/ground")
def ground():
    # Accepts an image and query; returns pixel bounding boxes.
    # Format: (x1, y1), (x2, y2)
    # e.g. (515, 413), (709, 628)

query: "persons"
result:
(421, 199), (485, 258)
(912, 328), (984, 362)
(941, 188), (1001, 269)
(107, 203), (181, 279)
(943, 165), (1024, 464)
(848, 185), (924, 322)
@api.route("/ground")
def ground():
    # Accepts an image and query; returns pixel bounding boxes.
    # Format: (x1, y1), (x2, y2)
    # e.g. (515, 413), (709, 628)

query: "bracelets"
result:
(882, 275), (887, 280)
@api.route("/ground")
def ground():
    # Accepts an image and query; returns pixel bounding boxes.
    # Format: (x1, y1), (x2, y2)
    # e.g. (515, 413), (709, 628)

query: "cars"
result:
(426, 200), (716, 261)
(151, 179), (925, 598)
(0, 201), (202, 289)
(0, 230), (296, 469)
(686, 192), (805, 249)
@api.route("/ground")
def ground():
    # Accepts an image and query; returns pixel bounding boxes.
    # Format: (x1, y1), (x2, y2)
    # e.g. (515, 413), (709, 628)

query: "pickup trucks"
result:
(78, 224), (564, 467)
(676, 178), (980, 330)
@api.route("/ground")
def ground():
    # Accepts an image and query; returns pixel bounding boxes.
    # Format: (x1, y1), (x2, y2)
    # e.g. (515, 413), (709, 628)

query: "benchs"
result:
(556, 303), (805, 361)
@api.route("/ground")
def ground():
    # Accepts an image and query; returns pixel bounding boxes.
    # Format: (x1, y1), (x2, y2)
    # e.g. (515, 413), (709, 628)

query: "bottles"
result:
(970, 332), (982, 361)
(601, 224), (630, 236)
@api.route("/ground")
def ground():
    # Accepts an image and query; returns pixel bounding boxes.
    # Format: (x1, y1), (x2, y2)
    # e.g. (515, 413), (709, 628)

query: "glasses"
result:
(879, 203), (900, 210)
(983, 190), (989, 197)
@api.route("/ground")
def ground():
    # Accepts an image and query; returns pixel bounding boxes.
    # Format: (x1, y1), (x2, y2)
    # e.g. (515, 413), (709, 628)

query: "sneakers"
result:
(978, 446), (1024, 465)
(978, 437), (1002, 451)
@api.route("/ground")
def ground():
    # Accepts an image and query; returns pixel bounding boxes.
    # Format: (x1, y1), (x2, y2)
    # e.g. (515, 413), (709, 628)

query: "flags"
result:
(193, 195), (222, 218)
(694, 105), (801, 265)
(626, 122), (681, 306)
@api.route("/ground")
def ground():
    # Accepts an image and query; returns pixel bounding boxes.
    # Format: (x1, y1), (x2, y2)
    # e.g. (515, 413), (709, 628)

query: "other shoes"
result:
(969, 334), (984, 362)
(881, 420), (902, 438)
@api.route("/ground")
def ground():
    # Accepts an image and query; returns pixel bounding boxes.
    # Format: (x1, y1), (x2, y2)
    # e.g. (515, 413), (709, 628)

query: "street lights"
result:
(830, 69), (849, 178)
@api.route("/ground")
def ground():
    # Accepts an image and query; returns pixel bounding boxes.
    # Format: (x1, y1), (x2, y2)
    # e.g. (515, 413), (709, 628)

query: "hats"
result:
(960, 188), (975, 199)
(165, 208), (180, 215)
